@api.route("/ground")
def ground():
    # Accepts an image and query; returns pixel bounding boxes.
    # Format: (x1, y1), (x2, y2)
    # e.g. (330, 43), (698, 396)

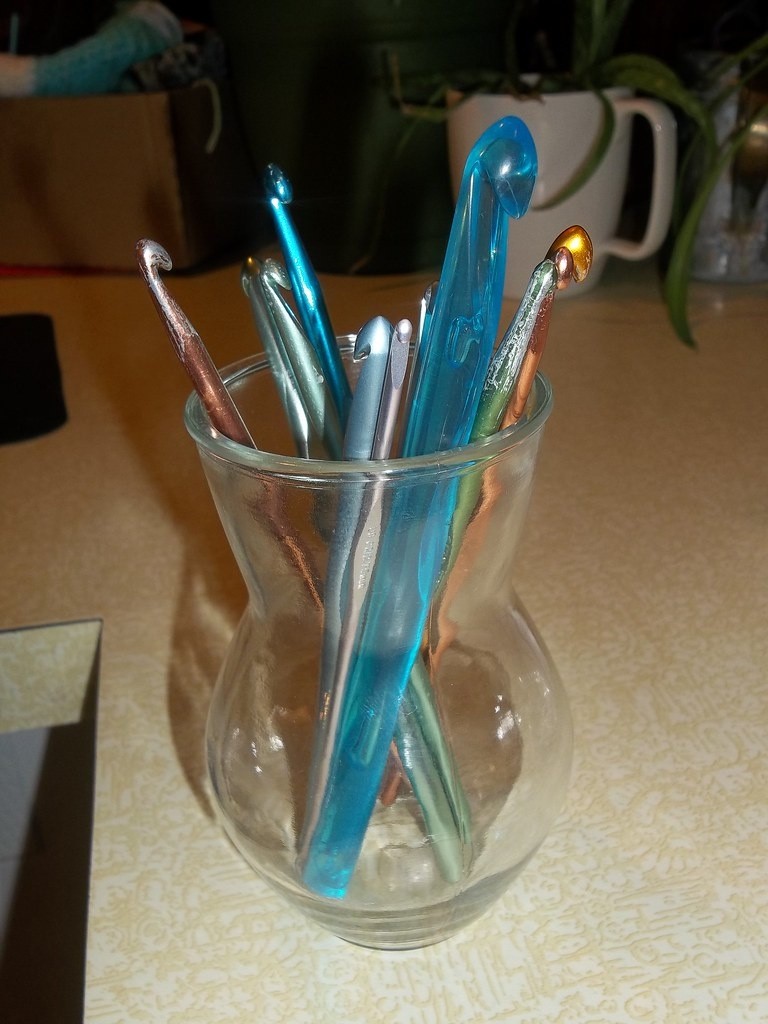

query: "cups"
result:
(670, 51), (767, 285)
(177, 323), (577, 950)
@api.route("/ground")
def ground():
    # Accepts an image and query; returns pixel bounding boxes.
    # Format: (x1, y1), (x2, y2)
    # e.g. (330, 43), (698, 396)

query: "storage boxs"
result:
(0, 70), (247, 271)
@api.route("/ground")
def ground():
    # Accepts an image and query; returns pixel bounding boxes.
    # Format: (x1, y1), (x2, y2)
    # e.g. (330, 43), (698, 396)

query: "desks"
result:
(0, 221), (768, 1024)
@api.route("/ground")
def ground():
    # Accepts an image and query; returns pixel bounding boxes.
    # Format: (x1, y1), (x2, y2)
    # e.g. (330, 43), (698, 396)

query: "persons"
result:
(1, 1), (214, 229)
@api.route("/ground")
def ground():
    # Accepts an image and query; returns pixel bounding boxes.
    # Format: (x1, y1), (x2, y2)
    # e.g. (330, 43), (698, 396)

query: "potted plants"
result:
(346, 0), (724, 299)
